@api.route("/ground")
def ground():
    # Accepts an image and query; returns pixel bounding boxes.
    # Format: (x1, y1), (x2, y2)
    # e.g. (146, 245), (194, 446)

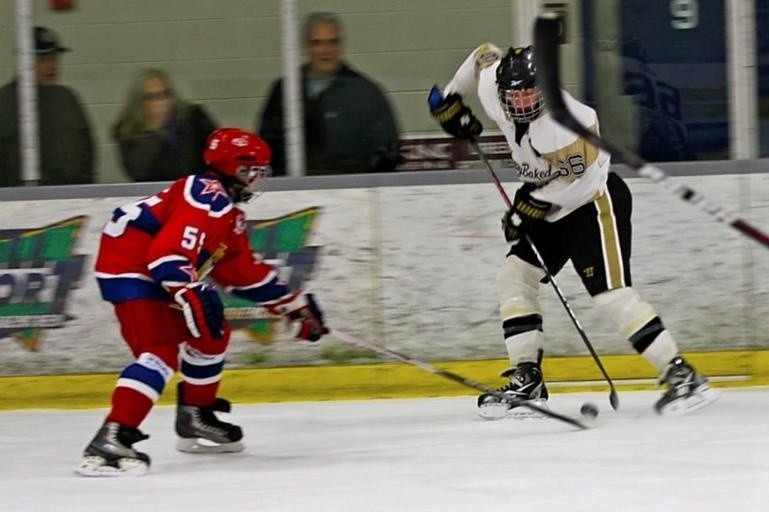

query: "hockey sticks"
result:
(532, 11), (769, 245)
(469, 136), (620, 412)
(325, 328), (599, 430)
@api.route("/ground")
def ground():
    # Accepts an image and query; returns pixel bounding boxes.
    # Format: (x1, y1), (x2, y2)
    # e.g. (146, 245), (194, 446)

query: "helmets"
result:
(495, 44), (544, 123)
(200, 127), (275, 206)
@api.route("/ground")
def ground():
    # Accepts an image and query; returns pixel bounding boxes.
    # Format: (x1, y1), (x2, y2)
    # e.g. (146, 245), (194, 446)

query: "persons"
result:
(114, 67), (216, 181)
(259, 12), (399, 174)
(433, 43), (699, 415)
(84, 124), (329, 465)
(0, 28), (94, 183)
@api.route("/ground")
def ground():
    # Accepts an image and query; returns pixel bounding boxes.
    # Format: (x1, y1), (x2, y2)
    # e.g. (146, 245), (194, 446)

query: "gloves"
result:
(425, 85), (484, 143)
(498, 179), (552, 243)
(286, 293), (330, 342)
(172, 279), (228, 342)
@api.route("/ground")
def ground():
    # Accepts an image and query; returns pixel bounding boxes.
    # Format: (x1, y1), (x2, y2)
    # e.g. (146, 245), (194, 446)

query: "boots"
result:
(81, 417), (153, 469)
(173, 380), (246, 444)
(651, 354), (710, 416)
(475, 361), (549, 409)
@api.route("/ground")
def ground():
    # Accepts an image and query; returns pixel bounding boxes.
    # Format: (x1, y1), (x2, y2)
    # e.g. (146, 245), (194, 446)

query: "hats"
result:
(31, 26), (71, 56)
